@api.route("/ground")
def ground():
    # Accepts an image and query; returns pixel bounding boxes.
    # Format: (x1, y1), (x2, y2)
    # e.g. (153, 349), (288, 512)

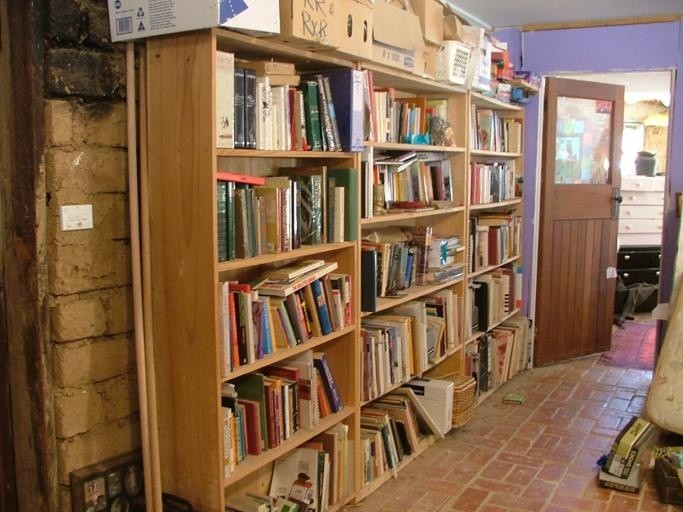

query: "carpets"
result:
(596, 321), (657, 371)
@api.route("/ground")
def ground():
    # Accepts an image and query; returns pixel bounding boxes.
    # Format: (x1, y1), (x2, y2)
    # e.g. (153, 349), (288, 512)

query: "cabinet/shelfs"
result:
(466, 89), (526, 409)
(138, 30), (359, 512)
(357, 59), (468, 499)
(614, 245), (662, 315)
(616, 175), (665, 253)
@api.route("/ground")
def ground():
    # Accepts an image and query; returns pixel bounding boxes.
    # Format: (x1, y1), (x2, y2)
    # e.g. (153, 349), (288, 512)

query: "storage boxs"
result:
(321, 0), (375, 61)
(424, 41), (439, 79)
(445, 15), (475, 46)
(470, 40), (492, 91)
(108, 0), (280, 44)
(372, 0), (425, 78)
(491, 51), (508, 77)
(278, 0), (340, 52)
(514, 70), (542, 84)
(410, 0), (443, 47)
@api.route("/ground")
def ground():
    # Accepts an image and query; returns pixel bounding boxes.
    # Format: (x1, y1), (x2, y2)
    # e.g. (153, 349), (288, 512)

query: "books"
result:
(469, 160), (533, 398)
(361, 289), (462, 486)
(367, 69), (460, 146)
(467, 104), (524, 152)
(223, 259), (350, 379)
(357, 225), (466, 311)
(361, 152), (454, 219)
(221, 349), (355, 512)
(217, 164), (357, 261)
(216, 50), (365, 155)
